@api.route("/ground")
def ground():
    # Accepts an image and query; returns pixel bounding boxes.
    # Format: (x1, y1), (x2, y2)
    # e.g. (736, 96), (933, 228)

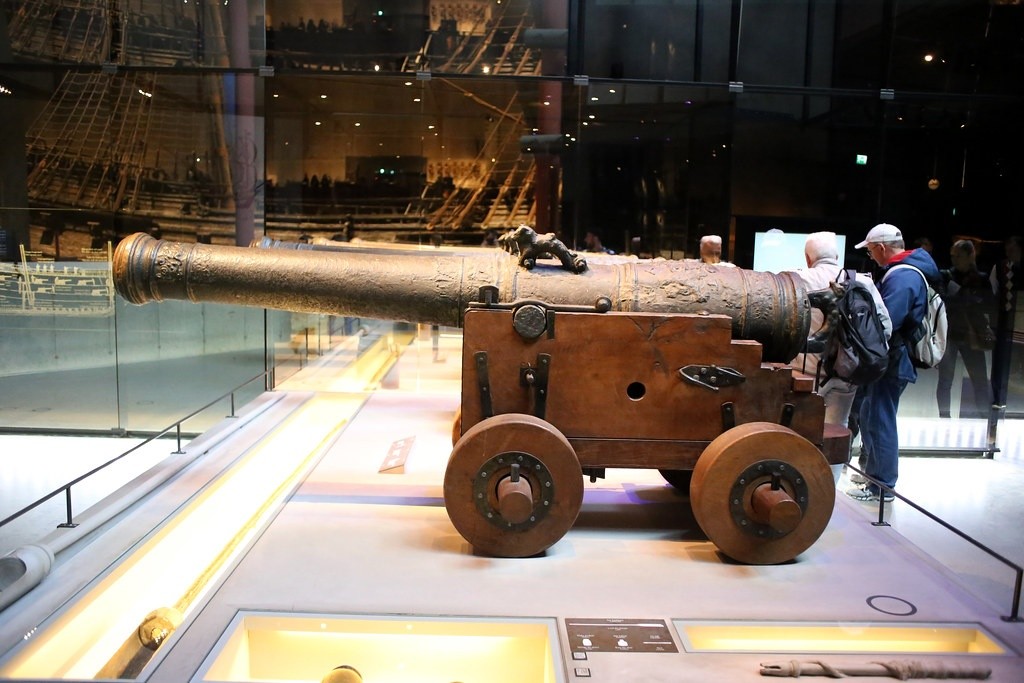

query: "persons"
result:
(847, 224), (939, 502)
(582, 229), (615, 255)
(327, 220), (353, 336)
(774, 233), (892, 483)
(935, 237), (1024, 417)
(680, 236), (733, 266)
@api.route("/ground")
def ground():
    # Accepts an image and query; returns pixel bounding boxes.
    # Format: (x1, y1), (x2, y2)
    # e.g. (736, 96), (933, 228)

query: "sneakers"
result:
(851, 473), (871, 484)
(845, 486), (895, 503)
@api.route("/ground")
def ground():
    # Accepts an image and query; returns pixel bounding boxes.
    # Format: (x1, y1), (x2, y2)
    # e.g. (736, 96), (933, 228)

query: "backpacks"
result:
(882, 265), (947, 369)
(821, 268), (889, 387)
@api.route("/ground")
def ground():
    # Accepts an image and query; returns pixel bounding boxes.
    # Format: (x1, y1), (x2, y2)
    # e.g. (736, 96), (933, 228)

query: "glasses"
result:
(865, 244), (885, 257)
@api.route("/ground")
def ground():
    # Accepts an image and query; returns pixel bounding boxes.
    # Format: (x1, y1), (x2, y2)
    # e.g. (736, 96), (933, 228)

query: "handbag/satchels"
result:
(968, 314), (996, 351)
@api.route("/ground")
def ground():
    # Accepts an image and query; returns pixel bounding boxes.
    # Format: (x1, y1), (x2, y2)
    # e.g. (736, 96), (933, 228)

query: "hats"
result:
(854, 223), (903, 249)
